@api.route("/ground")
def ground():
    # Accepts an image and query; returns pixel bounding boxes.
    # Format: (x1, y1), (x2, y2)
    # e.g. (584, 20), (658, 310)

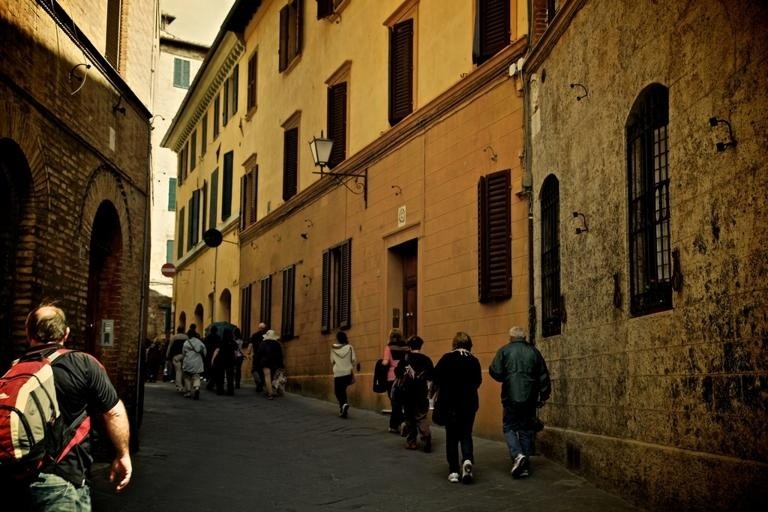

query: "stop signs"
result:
(161, 263), (177, 278)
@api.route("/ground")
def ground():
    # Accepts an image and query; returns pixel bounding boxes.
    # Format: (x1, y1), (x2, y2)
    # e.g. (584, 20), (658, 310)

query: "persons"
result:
(381, 329), (408, 435)
(145, 318), (287, 403)
(431, 332), (483, 483)
(329, 333), (356, 417)
(0, 294), (134, 511)
(486, 327), (551, 477)
(394, 335), (435, 452)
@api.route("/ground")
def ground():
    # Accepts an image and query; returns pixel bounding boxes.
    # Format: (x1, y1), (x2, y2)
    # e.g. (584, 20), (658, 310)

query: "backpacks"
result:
(0, 349), (106, 480)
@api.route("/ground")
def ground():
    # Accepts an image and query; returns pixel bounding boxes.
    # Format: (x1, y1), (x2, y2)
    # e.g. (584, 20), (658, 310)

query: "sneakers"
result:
(263, 393), (273, 400)
(184, 392), (191, 397)
(448, 472), (460, 482)
(194, 390), (199, 400)
(401, 422), (409, 437)
(510, 452), (529, 476)
(343, 403), (349, 417)
(462, 460), (474, 485)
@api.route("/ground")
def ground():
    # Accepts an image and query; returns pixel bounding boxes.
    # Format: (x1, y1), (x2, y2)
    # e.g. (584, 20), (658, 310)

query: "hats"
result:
(262, 329), (281, 341)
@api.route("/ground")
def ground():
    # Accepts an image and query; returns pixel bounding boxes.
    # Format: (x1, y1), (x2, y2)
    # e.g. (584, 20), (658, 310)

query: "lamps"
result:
(307, 130), (367, 208)
